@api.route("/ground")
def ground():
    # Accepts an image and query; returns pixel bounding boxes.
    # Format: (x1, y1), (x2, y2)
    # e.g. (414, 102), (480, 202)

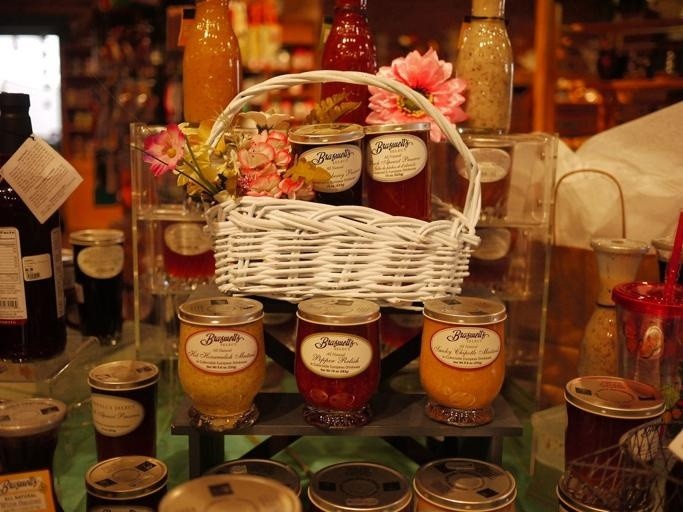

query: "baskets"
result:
(198, 68), (483, 310)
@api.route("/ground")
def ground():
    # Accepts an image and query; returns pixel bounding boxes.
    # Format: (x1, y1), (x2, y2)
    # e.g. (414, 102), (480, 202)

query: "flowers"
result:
(359, 42), (470, 145)
(96, 85), (365, 205)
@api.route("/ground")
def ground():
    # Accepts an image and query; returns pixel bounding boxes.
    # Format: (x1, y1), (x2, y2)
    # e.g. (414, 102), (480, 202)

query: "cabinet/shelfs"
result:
(161, 282), (530, 481)
(124, 118), (560, 408)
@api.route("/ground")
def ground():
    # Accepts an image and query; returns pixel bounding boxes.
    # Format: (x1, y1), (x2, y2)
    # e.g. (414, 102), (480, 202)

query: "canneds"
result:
(288, 122), (365, 220)
(305, 462), (413, 511)
(68, 228), (126, 347)
(555, 279), (683, 511)
(158, 473), (303, 510)
(363, 122), (432, 223)
(204, 459), (302, 497)
(419, 295), (507, 428)
(86, 360), (161, 462)
(159, 221), (216, 278)
(0, 397), (69, 512)
(447, 133), (514, 220)
(294, 296), (382, 431)
(177, 296), (267, 434)
(86, 457), (168, 510)
(412, 458), (518, 510)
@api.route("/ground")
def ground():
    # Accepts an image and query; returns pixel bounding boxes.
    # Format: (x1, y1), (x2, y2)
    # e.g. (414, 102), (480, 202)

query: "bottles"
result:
(320, 1), (378, 128)
(181, 1), (243, 127)
(652, 233), (683, 285)
(452, 1), (515, 136)
(575, 236), (649, 376)
(0, 93), (67, 361)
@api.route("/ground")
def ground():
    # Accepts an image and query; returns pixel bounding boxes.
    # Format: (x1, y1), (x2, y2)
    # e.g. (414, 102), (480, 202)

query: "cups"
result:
(611, 280), (682, 437)
